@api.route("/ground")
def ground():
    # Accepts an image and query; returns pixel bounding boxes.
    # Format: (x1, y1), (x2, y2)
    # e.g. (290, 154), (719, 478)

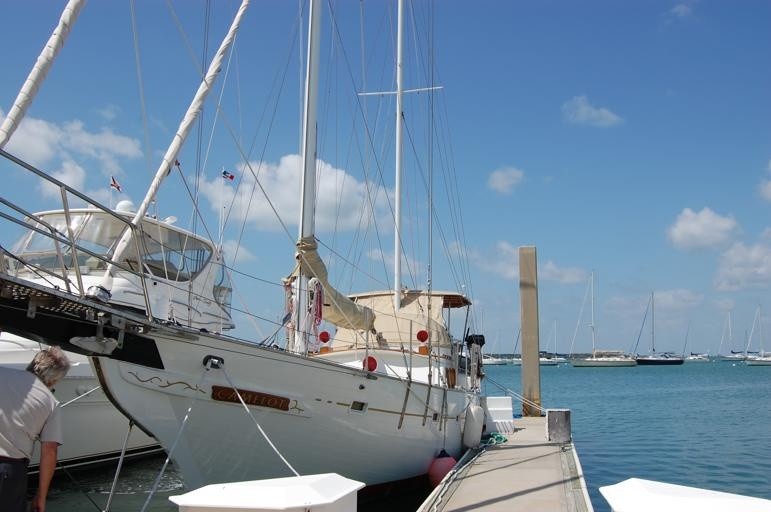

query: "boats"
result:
(1, 198), (235, 475)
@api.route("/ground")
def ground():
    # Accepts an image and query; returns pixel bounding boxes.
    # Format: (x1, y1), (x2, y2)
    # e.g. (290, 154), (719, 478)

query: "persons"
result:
(1, 345), (73, 511)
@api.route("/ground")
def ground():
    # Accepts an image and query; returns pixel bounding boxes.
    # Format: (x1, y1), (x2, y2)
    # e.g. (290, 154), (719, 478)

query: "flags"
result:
(110, 176), (122, 193)
(223, 170), (235, 182)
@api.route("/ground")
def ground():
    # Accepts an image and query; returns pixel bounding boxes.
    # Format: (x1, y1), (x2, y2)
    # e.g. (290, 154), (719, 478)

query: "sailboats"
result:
(481, 268), (771, 368)
(0, 1), (486, 493)
